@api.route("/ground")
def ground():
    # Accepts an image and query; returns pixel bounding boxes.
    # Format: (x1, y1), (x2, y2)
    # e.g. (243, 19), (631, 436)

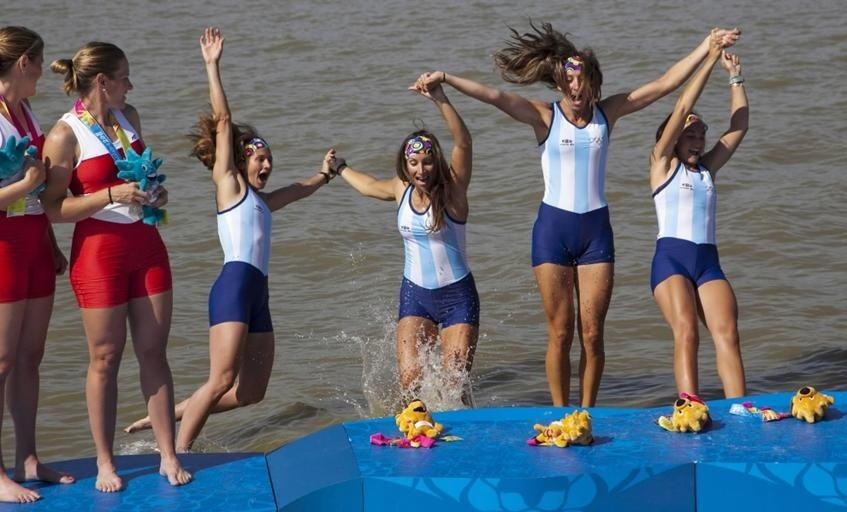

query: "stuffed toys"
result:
(117, 144), (168, 227)
(791, 385), (834, 425)
(0, 134), (50, 198)
(392, 397), (443, 442)
(526, 410), (595, 447)
(657, 392), (710, 433)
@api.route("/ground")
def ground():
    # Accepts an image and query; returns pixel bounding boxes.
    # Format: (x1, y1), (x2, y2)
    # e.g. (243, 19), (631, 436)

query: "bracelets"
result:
(729, 75), (745, 85)
(442, 72), (446, 81)
(107, 185), (115, 207)
(335, 162), (349, 175)
(318, 171), (326, 182)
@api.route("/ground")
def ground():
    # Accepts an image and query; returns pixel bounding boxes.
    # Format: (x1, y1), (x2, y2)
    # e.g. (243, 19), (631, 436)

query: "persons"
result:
(1, 25), (80, 504)
(333, 75), (482, 407)
(416, 15), (739, 407)
(122, 22), (342, 457)
(39, 41), (192, 493)
(650, 24), (752, 402)
